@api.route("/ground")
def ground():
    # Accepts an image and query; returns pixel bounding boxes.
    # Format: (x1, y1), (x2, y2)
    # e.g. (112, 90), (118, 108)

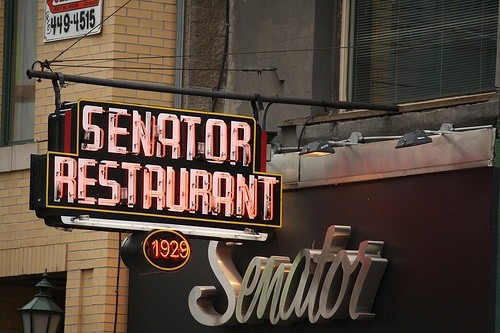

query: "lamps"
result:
(298, 140), (358, 157)
